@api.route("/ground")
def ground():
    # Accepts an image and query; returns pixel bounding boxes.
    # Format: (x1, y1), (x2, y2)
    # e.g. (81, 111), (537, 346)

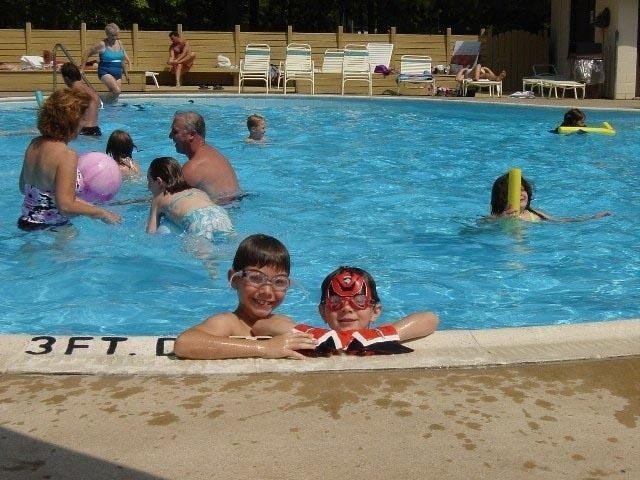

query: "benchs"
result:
(1, 70), (147, 92)
(150, 63), (240, 85)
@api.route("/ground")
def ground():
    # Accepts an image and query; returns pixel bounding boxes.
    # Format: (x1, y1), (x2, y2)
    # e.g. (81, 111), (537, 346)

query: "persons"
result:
(244, 113), (266, 142)
(455, 63), (506, 82)
(167, 31), (196, 87)
(174, 234), (319, 361)
(60, 62), (102, 137)
(106, 128), (145, 183)
(252, 266), (440, 352)
(477, 172), (612, 226)
(17, 87), (122, 232)
(146, 157), (236, 243)
(169, 110), (240, 208)
(555, 109), (586, 132)
(78, 23), (131, 104)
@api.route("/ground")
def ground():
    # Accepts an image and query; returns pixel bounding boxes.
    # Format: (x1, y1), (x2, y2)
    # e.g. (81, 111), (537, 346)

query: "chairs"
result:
(433, 40), (482, 97)
(396, 54), (436, 96)
(314, 49), (345, 74)
(521, 63), (585, 99)
(341, 44), (372, 96)
(366, 43), (395, 75)
(461, 78), (503, 97)
(238, 43), (272, 94)
(283, 42), (315, 95)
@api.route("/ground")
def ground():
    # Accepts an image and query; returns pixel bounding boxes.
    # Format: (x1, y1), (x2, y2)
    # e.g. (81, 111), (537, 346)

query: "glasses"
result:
(323, 273), (377, 311)
(229, 270), (290, 291)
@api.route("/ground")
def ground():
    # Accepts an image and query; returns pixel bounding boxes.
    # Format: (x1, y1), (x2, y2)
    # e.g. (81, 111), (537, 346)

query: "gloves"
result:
(293, 324), (414, 357)
